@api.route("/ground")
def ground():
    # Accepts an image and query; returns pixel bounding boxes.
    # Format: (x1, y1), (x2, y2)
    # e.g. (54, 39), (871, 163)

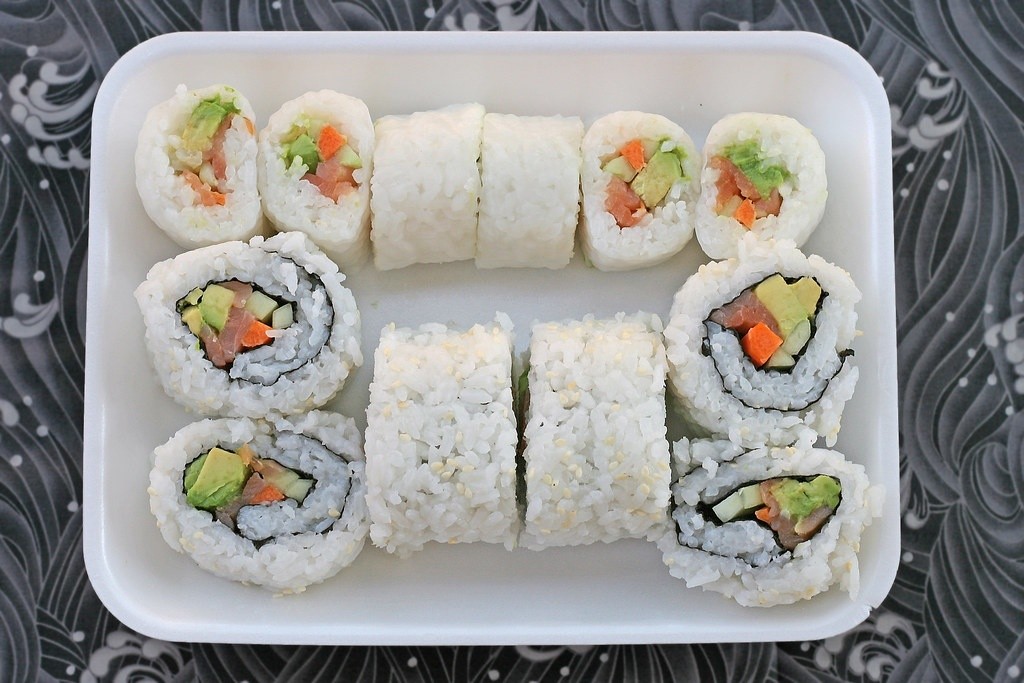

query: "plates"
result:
(83, 31), (901, 645)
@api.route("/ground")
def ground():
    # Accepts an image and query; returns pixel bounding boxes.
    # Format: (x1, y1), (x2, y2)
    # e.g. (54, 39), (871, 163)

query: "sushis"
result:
(135, 84), (886, 609)
